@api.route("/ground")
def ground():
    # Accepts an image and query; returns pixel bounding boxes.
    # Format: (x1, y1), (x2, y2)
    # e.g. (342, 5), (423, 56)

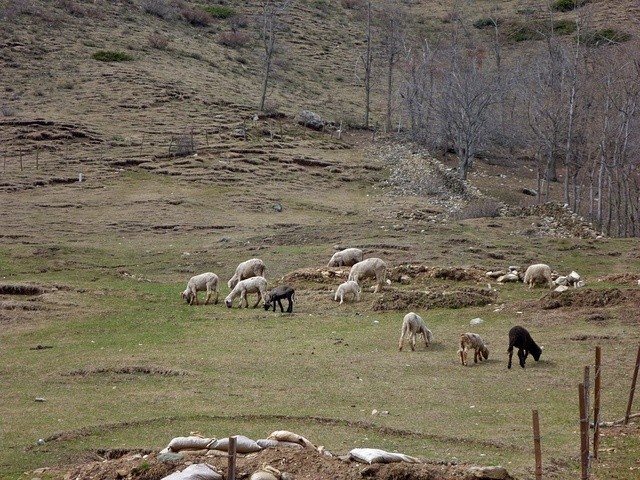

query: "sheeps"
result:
(263, 286), (296, 313)
(524, 264), (553, 289)
(182, 271), (219, 306)
(457, 333), (489, 366)
(507, 326), (543, 370)
(347, 258), (387, 293)
(398, 312), (433, 350)
(224, 276), (268, 309)
(226, 259), (267, 289)
(326, 248), (363, 267)
(334, 281), (362, 304)
